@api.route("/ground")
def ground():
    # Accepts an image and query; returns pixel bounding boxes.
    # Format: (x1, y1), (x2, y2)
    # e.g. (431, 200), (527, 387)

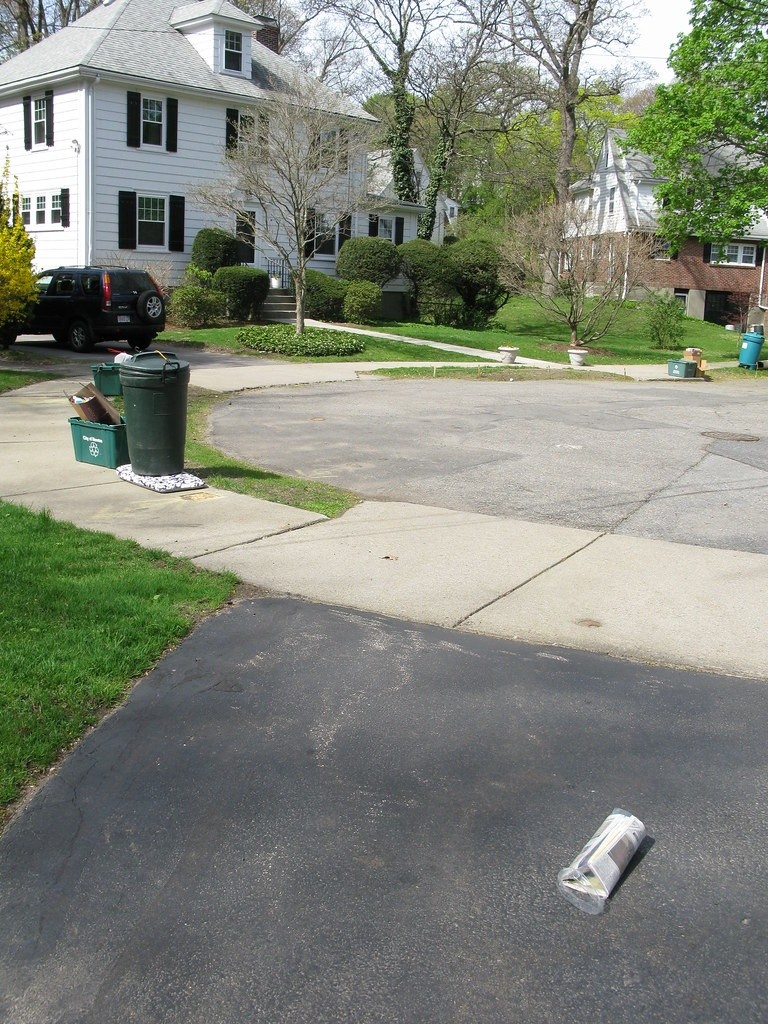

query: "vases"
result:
(498, 346), (519, 364)
(567, 349), (589, 367)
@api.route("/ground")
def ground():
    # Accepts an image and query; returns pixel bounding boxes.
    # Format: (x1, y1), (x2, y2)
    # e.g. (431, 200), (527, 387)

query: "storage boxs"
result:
(667, 347), (707, 378)
(90, 362), (124, 397)
(66, 415), (130, 470)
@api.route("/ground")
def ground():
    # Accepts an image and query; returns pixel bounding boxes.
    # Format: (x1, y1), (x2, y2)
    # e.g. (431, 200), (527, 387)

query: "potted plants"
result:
(271, 273), (282, 289)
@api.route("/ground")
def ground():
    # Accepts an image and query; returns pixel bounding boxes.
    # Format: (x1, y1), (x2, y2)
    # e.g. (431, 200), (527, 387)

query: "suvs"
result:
(11, 264), (167, 353)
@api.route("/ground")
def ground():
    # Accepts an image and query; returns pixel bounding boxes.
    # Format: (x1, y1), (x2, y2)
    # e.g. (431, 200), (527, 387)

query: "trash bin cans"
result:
(90, 362), (124, 397)
(119, 351), (190, 477)
(738, 331), (765, 369)
(667, 359), (696, 378)
(68, 416), (130, 469)
